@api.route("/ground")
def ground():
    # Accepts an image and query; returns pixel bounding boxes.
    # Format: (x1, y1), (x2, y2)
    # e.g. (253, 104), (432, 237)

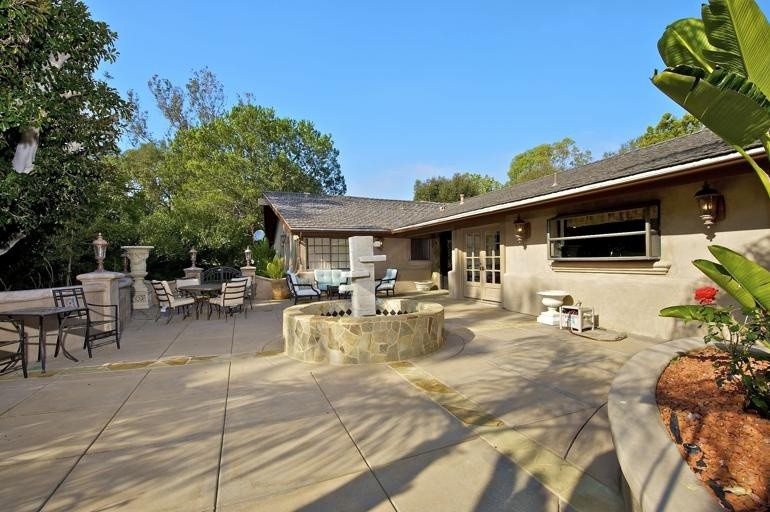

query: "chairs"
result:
(0, 286), (120, 378)
(150, 268), (399, 324)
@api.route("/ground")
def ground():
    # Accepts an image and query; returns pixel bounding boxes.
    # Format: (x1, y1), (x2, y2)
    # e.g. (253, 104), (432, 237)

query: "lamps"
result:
(512, 213), (531, 245)
(693, 181), (725, 230)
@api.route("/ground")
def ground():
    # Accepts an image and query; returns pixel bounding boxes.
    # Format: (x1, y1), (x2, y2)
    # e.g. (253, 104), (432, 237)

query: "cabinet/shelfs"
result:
(560, 305), (593, 332)
